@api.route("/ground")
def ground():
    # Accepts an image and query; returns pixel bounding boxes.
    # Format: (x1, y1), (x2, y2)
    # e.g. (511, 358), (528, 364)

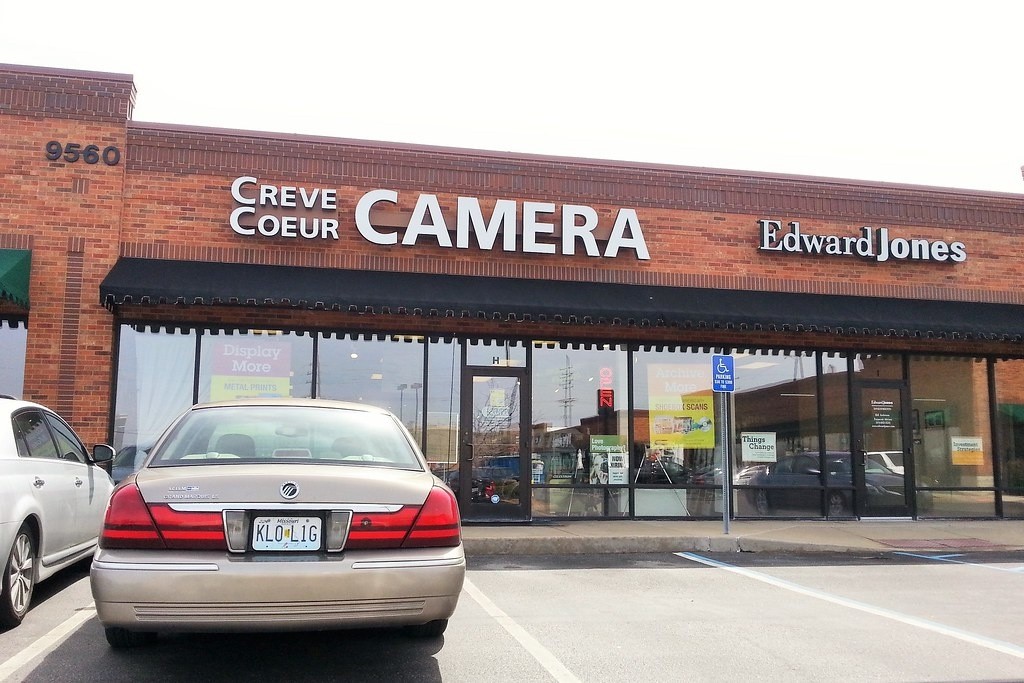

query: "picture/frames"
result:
(898, 408), (920, 432)
(925, 409), (945, 429)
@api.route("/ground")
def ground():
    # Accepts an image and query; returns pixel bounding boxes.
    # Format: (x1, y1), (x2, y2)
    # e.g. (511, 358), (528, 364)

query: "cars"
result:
(90, 398), (466, 648)
(634, 461), (691, 483)
(743, 451), (934, 516)
(0, 393), (115, 628)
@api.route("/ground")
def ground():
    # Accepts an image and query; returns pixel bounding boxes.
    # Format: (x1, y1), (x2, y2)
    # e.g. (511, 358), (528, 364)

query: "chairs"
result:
(330, 437), (369, 458)
(215, 434), (255, 457)
